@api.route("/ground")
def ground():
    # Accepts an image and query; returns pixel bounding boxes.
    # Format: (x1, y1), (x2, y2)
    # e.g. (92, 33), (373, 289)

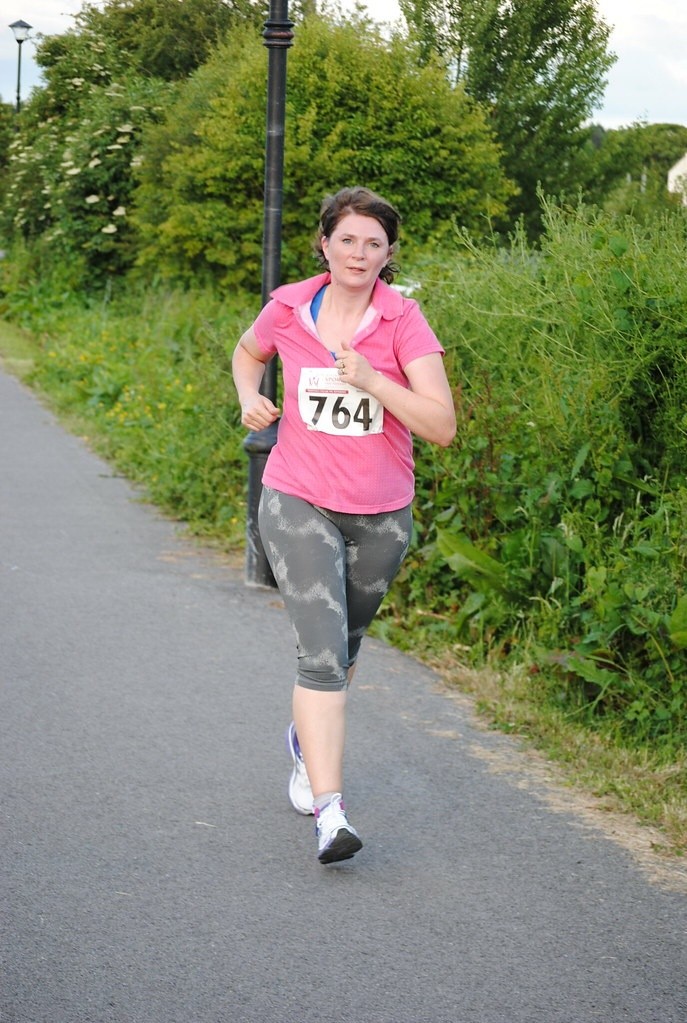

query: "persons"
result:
(233, 184), (456, 868)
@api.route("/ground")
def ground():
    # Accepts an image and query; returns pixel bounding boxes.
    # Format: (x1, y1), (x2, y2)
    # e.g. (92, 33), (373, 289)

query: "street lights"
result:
(9, 19), (33, 111)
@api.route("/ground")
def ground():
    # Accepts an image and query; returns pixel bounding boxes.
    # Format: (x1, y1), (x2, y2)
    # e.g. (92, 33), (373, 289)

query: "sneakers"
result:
(287, 720), (316, 814)
(315, 800), (363, 866)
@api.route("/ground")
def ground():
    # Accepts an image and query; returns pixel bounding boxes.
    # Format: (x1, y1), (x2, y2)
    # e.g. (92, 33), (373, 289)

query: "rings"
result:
(340, 359), (345, 368)
(342, 367), (345, 375)
